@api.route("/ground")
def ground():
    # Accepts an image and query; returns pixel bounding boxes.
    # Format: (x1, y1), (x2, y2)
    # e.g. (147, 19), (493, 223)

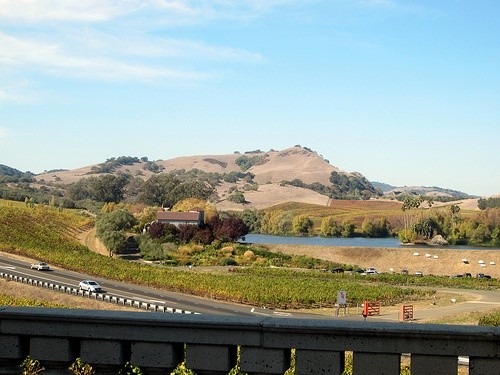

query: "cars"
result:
(79, 280), (101, 292)
(462, 258), (496, 267)
(413, 252), (439, 259)
(30, 261), (49, 270)
(332, 267), (491, 279)
